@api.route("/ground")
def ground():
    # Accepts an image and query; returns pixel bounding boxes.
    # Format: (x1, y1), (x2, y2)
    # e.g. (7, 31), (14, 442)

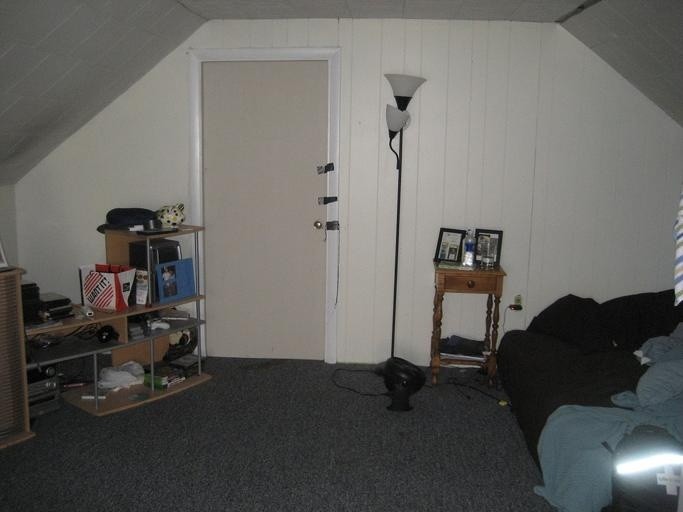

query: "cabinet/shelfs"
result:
(25, 224), (213, 418)
(0, 265), (36, 450)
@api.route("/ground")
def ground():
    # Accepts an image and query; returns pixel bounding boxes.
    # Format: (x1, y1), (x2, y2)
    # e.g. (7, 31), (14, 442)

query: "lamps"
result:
(375, 74), (427, 376)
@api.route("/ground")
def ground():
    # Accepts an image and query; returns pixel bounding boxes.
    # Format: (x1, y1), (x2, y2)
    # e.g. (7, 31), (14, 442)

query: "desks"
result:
(429, 258), (507, 385)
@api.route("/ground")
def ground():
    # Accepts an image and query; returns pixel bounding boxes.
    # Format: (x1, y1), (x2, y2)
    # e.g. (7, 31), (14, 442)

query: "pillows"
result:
(526, 288), (682, 357)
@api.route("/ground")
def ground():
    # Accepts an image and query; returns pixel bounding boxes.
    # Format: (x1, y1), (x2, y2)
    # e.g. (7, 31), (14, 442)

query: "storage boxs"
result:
(78, 264), (137, 312)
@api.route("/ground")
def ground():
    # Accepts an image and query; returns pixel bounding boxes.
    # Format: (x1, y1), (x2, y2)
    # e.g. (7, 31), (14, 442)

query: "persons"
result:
(162, 266), (176, 297)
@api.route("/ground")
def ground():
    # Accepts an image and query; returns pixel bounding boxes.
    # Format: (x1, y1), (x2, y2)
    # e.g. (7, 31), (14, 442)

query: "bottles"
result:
(462, 229), (475, 267)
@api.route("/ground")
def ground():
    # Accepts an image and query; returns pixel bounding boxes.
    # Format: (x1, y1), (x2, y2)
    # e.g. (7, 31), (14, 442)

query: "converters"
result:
(509, 304), (523, 311)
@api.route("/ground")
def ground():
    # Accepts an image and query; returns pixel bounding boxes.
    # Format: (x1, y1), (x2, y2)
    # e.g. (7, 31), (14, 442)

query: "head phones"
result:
(96, 325), (120, 343)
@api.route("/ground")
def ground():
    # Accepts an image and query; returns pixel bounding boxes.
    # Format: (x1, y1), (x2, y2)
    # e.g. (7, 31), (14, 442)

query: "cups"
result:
(480, 238), (495, 270)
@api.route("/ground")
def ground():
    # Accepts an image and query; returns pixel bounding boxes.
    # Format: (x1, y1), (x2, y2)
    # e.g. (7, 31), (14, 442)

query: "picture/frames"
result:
(433, 227), (465, 263)
(472, 229), (503, 265)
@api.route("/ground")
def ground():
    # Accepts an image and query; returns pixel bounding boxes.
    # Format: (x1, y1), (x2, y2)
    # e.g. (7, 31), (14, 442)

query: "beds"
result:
(496, 328), (683, 512)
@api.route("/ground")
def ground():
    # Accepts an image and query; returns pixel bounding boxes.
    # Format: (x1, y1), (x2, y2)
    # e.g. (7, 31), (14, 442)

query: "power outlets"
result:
(511, 294), (525, 312)
